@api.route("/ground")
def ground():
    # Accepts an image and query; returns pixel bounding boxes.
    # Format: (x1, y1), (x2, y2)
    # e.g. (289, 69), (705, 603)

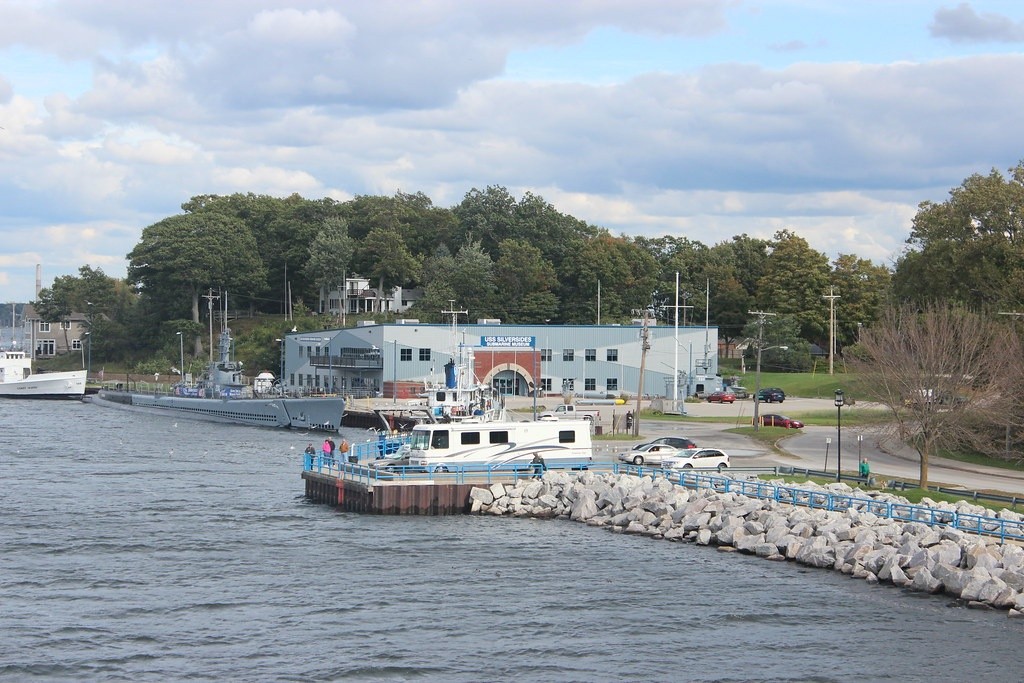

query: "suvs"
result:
(752, 387), (786, 403)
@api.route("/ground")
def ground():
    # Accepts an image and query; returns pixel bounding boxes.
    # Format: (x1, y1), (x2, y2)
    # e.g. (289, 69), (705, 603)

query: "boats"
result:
(372, 300), (507, 454)
(97, 292), (348, 434)
(0, 301), (89, 401)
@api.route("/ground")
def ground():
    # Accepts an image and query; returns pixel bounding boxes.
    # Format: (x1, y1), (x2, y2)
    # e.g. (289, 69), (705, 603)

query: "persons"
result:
(323, 440), (331, 465)
(305, 443), (315, 470)
(532, 451), (547, 479)
(857, 457), (869, 484)
(328, 437), (335, 467)
(626, 410), (633, 427)
(339, 438), (349, 463)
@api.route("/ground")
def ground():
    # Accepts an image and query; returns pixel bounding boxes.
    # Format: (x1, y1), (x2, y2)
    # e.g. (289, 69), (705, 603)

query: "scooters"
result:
(625, 416), (633, 429)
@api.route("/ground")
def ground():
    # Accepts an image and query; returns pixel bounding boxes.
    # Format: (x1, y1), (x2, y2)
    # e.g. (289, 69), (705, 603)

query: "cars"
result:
(370, 442), (435, 473)
(904, 388), (971, 415)
(660, 447), (730, 473)
(751, 413), (804, 430)
(707, 391), (736, 404)
(731, 387), (751, 399)
(631, 436), (698, 456)
(618, 443), (679, 465)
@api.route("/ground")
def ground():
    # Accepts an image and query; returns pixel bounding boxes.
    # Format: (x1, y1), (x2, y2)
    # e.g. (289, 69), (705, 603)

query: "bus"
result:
(409, 416), (594, 473)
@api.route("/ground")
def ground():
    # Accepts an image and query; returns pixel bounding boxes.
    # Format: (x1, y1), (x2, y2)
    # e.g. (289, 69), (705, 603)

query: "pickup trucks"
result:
(538, 404), (602, 425)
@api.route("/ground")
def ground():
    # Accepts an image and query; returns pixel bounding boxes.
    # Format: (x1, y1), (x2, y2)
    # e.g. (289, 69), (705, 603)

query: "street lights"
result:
(274, 338), (284, 387)
(85, 332), (92, 383)
(753, 344), (789, 432)
(176, 331), (185, 382)
(324, 337), (333, 394)
(388, 340), (397, 404)
(834, 388), (844, 483)
